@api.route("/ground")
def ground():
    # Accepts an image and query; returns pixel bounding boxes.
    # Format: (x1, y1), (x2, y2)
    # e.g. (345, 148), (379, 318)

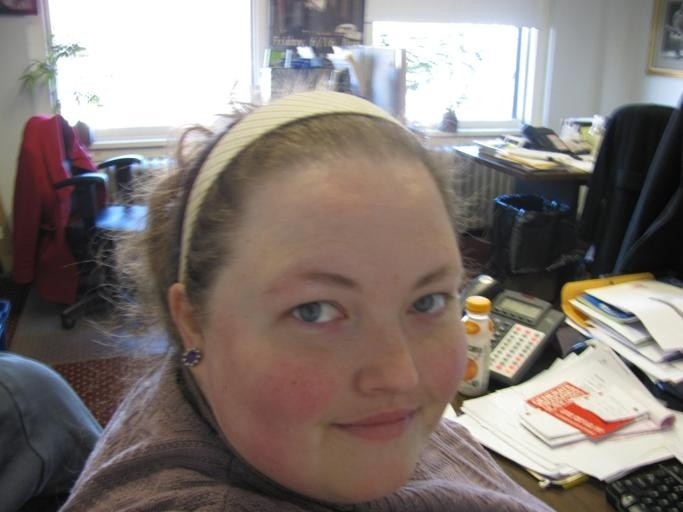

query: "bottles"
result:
(455, 295), (494, 398)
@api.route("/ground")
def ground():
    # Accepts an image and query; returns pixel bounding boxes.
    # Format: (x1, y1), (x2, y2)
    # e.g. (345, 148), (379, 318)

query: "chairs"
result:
(24, 113), (152, 326)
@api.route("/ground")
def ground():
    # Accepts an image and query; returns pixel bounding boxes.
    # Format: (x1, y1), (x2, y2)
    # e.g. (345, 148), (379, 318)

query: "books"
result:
(522, 391), (679, 453)
(471, 135), (571, 175)
(568, 275), (683, 389)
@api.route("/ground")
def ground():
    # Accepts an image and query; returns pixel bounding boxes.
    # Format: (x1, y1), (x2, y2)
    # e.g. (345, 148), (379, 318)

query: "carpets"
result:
(41, 349), (166, 431)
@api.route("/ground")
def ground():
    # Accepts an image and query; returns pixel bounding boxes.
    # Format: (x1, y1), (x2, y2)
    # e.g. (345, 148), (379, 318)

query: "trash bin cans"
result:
(491, 195), (570, 278)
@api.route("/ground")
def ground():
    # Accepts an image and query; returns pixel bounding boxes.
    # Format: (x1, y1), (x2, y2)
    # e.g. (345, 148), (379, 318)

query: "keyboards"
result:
(604, 455), (683, 510)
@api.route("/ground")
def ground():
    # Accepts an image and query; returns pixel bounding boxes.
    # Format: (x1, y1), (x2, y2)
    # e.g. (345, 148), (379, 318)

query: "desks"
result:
(441, 263), (682, 511)
(452, 139), (596, 280)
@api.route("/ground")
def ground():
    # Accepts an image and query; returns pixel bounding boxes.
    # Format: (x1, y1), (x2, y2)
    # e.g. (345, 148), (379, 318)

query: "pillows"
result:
(0, 351), (111, 511)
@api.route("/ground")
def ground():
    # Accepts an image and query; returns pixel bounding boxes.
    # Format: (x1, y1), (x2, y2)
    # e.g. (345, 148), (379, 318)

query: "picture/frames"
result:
(643, 1), (683, 77)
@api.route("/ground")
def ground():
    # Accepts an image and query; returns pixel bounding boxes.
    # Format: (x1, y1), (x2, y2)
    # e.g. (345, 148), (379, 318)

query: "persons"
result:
(60, 91), (560, 511)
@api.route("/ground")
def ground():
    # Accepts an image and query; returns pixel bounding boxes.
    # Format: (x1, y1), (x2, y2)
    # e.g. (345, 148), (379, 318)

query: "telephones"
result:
(458, 275), (566, 385)
(523, 126), (570, 152)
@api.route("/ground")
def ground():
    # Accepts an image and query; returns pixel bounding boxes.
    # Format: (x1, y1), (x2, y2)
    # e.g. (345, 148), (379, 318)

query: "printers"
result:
(559, 116), (593, 140)
